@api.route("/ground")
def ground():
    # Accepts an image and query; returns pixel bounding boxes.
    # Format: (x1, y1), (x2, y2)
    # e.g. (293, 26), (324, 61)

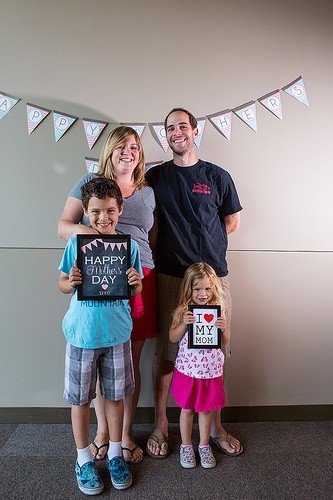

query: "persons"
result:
(58, 177), (144, 495)
(58, 125), (156, 463)
(143, 108), (244, 459)
(169, 264), (230, 468)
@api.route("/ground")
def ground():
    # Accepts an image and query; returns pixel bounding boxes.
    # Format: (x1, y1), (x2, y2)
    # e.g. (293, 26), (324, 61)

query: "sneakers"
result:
(198, 444), (216, 468)
(74, 460), (104, 495)
(105, 455), (133, 490)
(180, 444), (196, 469)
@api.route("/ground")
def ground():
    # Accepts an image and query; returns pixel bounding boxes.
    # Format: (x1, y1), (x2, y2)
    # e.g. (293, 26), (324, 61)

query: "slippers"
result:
(89, 441), (109, 460)
(121, 441), (144, 464)
(146, 423), (170, 458)
(210, 432), (244, 456)
